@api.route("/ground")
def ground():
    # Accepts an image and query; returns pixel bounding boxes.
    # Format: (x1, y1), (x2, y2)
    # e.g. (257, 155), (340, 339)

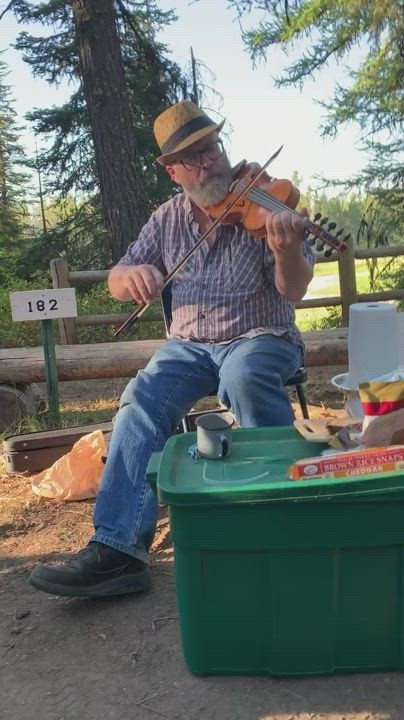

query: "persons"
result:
(27, 101), (315, 599)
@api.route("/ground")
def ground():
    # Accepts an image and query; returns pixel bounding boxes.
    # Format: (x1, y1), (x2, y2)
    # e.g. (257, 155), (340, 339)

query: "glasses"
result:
(170, 138), (225, 170)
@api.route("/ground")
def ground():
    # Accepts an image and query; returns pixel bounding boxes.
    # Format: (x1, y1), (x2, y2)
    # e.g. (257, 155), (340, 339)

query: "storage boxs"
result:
(156, 426), (404, 679)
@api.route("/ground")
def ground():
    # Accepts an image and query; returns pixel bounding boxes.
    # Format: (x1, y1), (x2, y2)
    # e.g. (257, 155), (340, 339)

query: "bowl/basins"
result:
(330, 370), (392, 401)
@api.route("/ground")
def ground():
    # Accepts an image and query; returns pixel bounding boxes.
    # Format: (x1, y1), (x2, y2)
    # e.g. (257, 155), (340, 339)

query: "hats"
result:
(153, 100), (226, 167)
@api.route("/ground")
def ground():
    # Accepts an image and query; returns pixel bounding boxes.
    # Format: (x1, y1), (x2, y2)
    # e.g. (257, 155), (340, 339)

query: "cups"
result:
(194, 413), (235, 460)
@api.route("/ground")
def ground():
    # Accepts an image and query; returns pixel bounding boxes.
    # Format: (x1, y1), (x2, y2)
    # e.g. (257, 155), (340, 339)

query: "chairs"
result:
(160, 288), (309, 433)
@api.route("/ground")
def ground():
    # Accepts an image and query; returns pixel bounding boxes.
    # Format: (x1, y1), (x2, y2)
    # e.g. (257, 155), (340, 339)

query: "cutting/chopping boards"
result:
(293, 417), (365, 442)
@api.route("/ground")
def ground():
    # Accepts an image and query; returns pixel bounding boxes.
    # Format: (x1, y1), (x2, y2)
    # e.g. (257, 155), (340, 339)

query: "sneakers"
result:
(28, 543), (154, 598)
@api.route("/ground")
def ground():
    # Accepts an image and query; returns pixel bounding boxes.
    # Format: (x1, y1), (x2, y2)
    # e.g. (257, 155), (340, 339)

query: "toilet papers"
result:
(348, 302), (396, 385)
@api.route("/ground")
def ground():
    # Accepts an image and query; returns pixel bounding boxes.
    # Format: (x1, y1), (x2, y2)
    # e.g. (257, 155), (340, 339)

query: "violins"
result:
(206, 162), (352, 258)
